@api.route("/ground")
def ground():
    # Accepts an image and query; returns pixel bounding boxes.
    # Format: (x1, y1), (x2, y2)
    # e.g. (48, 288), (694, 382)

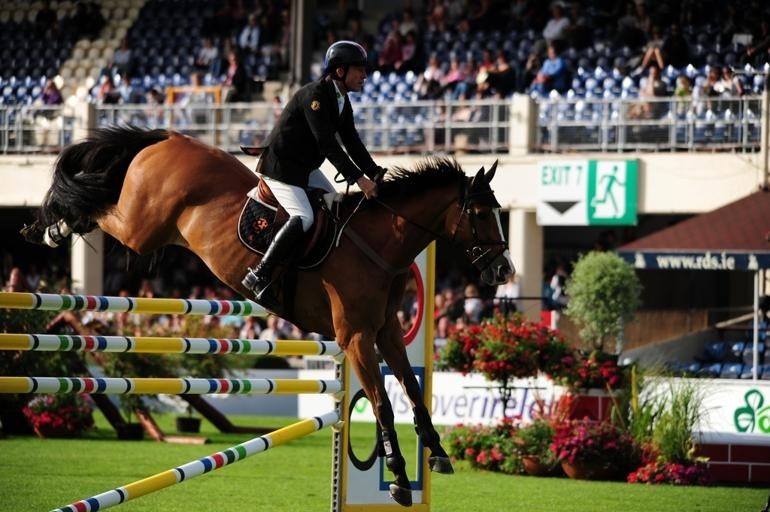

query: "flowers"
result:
(435, 311), (582, 384)
(22, 391), (82, 431)
(450, 384), (711, 486)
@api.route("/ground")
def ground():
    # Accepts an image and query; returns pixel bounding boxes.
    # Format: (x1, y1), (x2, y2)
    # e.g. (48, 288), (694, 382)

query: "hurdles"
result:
(0, 240), (436, 512)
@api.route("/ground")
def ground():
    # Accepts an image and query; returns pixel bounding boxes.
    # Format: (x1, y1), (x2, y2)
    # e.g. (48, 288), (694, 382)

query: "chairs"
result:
(669, 317), (770, 380)
(0, 0), (770, 155)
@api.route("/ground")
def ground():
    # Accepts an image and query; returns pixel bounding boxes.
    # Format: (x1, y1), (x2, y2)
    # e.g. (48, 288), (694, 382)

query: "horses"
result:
(14, 123), (517, 509)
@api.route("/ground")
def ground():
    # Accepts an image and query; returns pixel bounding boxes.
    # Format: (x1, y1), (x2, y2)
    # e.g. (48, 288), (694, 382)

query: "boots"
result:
(242, 217), (304, 306)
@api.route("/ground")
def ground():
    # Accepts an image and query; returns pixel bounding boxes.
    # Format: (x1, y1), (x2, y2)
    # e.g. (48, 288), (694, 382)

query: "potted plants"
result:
(104, 311), (252, 441)
(567, 311), (635, 426)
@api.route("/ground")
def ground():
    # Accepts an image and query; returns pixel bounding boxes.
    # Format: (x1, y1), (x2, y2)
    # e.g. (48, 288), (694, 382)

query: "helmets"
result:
(324, 40), (369, 70)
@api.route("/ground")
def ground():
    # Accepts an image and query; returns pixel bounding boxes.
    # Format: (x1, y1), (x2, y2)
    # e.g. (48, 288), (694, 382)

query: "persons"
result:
(398, 284), (483, 339)
(1, 265), (301, 372)
(192, 0), (290, 137)
(353, 2), (770, 148)
(541, 266), (570, 331)
(92, 38), (208, 127)
(31, 80), (64, 144)
(242, 41), (392, 300)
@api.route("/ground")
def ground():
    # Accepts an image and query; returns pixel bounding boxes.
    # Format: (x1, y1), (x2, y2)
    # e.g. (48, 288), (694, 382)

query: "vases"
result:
(34, 427), (68, 438)
